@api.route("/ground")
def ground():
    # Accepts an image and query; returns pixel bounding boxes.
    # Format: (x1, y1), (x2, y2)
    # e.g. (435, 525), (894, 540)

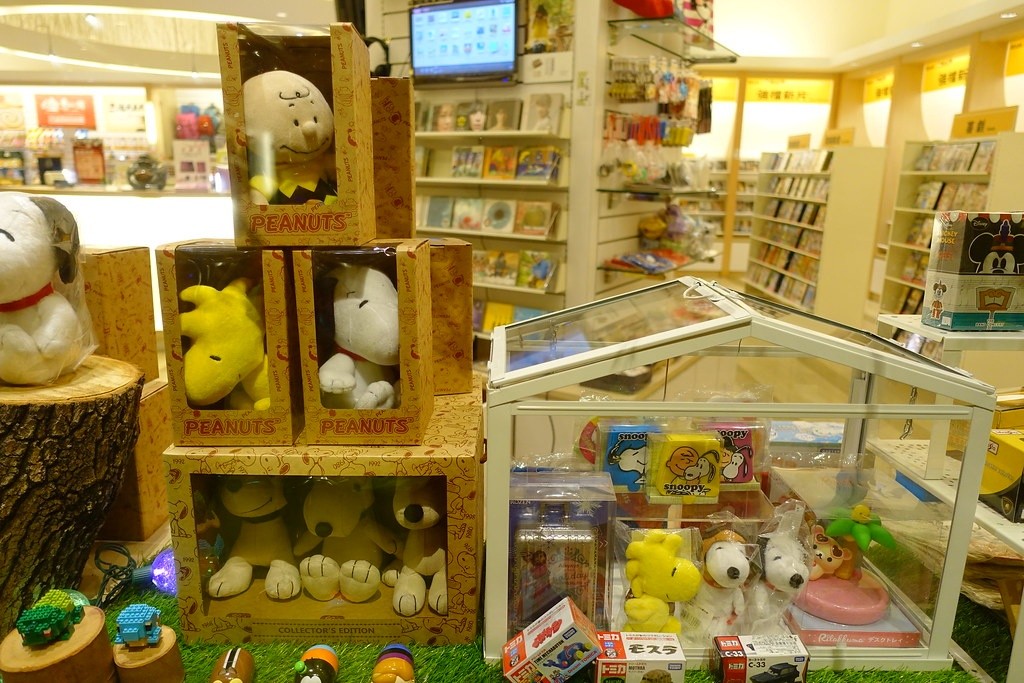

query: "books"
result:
(752, 151), (833, 310)
(891, 141), (996, 360)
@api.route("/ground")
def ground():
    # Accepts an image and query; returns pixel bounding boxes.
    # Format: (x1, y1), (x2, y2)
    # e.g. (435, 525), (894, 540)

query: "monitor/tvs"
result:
(409, 0), (519, 86)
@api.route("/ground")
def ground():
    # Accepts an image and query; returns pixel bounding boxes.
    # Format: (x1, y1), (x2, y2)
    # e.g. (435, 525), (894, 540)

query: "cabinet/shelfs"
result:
(876, 131), (1024, 393)
(412, 128), (571, 343)
(745, 145), (891, 330)
(593, 14), (738, 279)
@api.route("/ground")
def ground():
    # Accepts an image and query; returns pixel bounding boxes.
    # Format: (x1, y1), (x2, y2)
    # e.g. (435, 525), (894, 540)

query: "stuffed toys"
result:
(623, 520), (854, 642)
(315, 260), (400, 410)
(180, 276), (270, 409)
(243, 70), (337, 206)
(0, 197), (80, 384)
(206, 474), (448, 617)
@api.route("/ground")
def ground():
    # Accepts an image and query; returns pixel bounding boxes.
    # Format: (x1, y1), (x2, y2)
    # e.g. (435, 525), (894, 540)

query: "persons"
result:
(519, 549), (560, 621)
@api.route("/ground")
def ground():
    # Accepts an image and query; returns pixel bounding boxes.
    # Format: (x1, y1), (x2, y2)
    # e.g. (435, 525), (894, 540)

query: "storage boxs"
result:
(51, 22), (486, 646)
(482, 274), (1024, 683)
(919, 211), (1024, 333)
